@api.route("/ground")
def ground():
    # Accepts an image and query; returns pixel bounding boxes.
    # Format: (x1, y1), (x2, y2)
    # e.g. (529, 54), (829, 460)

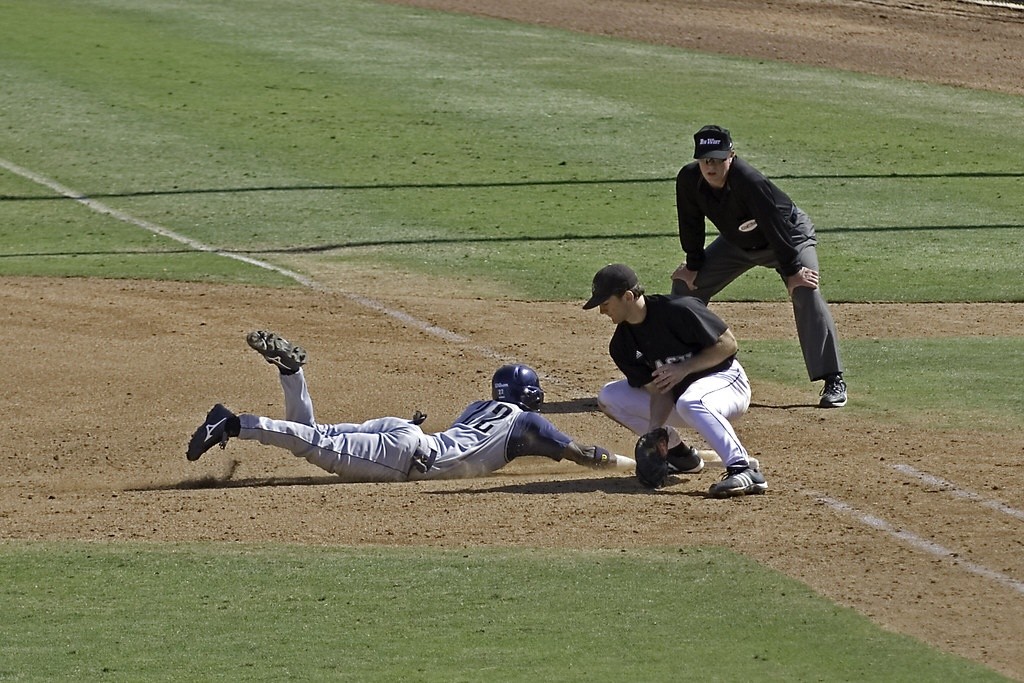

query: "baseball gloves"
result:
(635, 427), (669, 488)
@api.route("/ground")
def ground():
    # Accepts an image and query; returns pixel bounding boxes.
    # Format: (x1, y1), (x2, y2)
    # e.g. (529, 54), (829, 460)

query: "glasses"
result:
(701, 159), (724, 164)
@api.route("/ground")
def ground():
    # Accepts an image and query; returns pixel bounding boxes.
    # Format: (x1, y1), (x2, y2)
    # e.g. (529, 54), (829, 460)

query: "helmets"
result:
(492, 364), (544, 413)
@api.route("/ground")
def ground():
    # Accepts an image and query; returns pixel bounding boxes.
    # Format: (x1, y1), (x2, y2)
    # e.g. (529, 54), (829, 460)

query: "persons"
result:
(669, 125), (848, 407)
(583, 264), (768, 497)
(187, 330), (636, 482)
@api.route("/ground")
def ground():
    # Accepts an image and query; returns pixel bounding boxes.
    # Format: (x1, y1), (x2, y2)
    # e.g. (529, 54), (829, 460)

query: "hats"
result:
(693, 130), (733, 159)
(583, 264), (639, 310)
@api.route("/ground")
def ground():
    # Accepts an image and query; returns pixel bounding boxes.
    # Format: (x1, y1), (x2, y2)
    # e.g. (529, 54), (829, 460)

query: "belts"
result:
(425, 450), (437, 472)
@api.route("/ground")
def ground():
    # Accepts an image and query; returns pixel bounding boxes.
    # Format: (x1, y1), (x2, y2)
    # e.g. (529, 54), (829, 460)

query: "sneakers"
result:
(245, 330), (307, 371)
(818, 376), (847, 408)
(186, 404), (237, 462)
(709, 457), (769, 497)
(666, 446), (705, 474)
